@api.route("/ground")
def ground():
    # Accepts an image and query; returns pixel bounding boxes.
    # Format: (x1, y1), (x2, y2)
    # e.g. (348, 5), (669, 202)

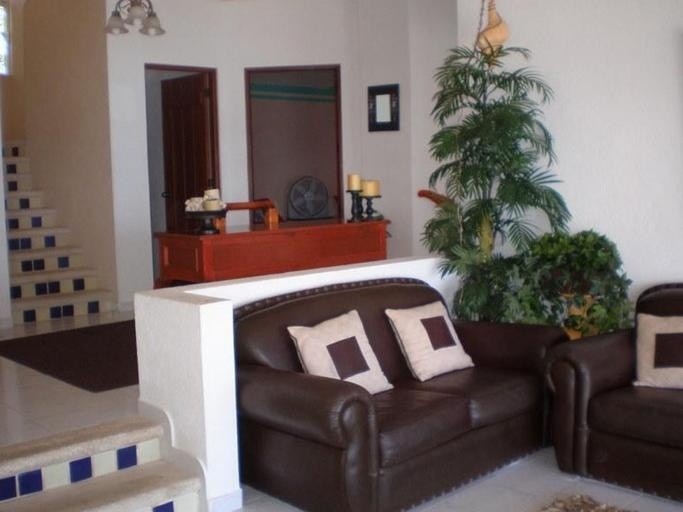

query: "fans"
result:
(288, 176), (329, 220)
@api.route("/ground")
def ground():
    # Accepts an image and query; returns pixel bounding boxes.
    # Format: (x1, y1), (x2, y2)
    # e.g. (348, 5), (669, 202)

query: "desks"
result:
(154, 218), (386, 287)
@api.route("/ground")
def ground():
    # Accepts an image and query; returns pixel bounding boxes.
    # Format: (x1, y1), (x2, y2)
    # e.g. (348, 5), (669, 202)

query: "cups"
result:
(204, 198), (226, 212)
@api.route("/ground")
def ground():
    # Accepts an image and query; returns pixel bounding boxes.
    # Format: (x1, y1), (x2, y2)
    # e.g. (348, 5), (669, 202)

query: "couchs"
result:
(233, 278), (562, 512)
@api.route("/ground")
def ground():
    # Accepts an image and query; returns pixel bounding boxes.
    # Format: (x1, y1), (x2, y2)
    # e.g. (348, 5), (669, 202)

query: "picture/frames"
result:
(368, 82), (398, 132)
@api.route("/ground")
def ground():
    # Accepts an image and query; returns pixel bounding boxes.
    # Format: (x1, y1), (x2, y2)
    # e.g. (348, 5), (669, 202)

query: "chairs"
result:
(546, 282), (683, 502)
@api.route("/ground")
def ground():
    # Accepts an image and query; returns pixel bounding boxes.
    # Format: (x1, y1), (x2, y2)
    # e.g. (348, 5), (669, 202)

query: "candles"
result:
(360, 180), (380, 195)
(347, 174), (361, 190)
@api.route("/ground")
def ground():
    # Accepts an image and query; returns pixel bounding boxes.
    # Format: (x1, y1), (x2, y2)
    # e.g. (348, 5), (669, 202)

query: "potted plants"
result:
(505, 229), (639, 340)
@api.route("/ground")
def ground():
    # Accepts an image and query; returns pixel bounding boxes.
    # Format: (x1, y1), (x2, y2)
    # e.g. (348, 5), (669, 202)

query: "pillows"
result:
(632, 313), (683, 390)
(385, 300), (475, 384)
(288, 309), (394, 396)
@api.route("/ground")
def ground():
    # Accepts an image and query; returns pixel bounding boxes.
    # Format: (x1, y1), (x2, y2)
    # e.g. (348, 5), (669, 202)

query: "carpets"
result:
(0, 320), (139, 392)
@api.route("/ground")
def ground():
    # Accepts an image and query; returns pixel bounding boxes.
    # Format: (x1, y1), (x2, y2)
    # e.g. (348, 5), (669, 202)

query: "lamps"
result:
(104, 0), (165, 36)
